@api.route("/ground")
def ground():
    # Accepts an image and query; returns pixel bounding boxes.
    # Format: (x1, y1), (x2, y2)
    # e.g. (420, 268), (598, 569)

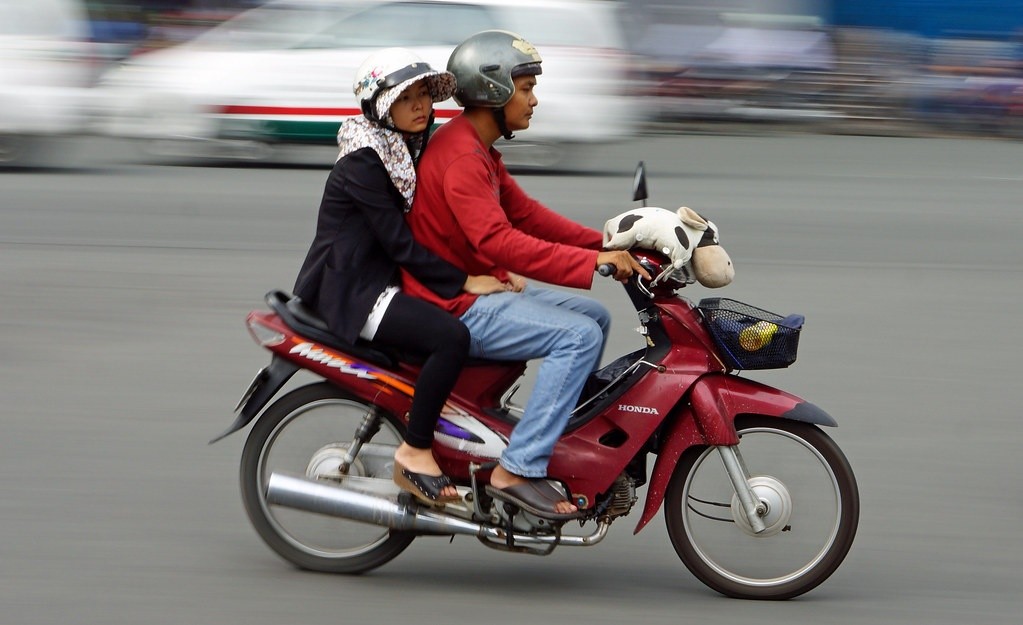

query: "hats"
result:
(353, 48), (458, 126)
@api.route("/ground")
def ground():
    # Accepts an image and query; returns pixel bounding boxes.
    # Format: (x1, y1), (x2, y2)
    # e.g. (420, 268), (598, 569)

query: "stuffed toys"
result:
(601, 206), (734, 287)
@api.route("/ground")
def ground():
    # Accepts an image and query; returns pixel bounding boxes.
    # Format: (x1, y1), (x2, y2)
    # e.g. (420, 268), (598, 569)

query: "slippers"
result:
(485, 473), (582, 520)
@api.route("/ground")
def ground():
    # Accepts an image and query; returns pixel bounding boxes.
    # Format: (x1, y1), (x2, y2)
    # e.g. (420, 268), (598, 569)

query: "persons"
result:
(403, 30), (651, 515)
(291, 47), (527, 504)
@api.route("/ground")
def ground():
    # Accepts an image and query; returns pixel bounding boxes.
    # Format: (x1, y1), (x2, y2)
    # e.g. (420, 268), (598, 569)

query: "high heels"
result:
(392, 462), (463, 505)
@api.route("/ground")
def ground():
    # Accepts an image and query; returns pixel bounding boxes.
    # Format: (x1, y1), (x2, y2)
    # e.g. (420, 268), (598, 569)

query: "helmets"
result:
(447, 30), (542, 108)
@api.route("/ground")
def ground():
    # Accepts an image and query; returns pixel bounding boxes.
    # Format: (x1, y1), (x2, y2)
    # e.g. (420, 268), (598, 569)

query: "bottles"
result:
(740, 321), (778, 351)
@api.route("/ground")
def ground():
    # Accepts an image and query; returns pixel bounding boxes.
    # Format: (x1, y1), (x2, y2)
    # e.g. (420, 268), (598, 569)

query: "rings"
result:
(517, 278), (522, 281)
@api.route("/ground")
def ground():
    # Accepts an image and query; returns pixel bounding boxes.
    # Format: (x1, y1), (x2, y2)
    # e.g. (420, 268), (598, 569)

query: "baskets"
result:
(698, 297), (803, 370)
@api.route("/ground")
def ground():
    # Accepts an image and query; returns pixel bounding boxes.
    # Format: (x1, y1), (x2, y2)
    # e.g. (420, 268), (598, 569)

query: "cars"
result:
(93, 0), (637, 145)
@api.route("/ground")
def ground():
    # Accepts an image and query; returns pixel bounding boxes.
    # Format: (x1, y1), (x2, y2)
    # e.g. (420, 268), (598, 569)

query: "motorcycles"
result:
(209, 161), (860, 599)
